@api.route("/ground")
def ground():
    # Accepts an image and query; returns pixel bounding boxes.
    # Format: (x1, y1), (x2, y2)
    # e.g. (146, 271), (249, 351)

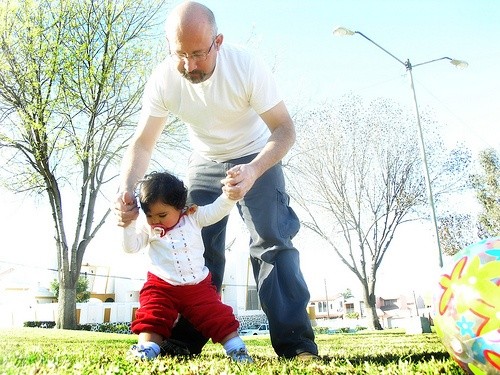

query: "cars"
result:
(240, 323), (270, 335)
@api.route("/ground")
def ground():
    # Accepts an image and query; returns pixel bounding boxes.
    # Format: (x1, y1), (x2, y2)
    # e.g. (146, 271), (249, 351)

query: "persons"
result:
(120, 168), (256, 365)
(113, 0), (322, 363)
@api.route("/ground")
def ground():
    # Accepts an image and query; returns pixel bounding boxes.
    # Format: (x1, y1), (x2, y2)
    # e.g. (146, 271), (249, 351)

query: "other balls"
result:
(433, 236), (500, 375)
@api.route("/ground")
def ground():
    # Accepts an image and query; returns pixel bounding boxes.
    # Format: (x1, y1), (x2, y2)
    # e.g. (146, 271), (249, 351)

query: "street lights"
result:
(330, 26), (469, 269)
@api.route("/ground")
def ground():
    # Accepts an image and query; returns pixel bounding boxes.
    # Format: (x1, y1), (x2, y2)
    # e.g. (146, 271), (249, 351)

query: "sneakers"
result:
(230, 348), (253, 363)
(129, 344), (156, 362)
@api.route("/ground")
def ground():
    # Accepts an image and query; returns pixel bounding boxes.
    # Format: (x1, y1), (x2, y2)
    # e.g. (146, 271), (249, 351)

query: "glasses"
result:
(173, 41), (214, 61)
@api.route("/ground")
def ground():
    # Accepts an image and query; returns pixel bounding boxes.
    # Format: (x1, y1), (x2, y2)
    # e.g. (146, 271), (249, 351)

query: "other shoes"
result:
(294, 352), (319, 362)
